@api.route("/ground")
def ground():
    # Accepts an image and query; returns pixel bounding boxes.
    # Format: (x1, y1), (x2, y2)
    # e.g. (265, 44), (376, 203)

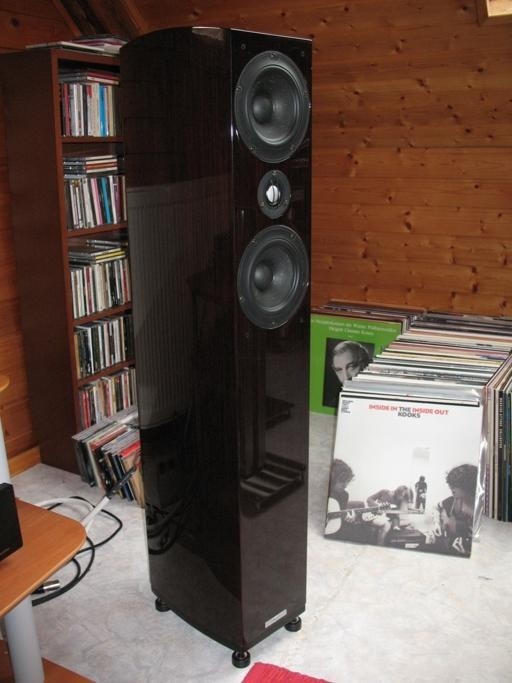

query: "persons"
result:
(330, 341), (371, 387)
(325, 460), (479, 559)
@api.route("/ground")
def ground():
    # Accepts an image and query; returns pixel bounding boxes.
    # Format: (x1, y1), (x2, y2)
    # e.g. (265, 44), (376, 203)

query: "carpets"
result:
(242, 662), (330, 683)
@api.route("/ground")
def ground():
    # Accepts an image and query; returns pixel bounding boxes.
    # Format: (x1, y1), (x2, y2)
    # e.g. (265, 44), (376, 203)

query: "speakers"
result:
(119, 26), (313, 651)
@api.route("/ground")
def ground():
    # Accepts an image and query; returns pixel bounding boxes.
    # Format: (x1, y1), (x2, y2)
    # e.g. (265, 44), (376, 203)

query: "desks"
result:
(2, 496), (88, 681)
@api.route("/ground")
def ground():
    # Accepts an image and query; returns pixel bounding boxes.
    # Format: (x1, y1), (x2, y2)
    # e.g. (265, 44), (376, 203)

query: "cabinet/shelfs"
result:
(0, 47), (130, 473)
